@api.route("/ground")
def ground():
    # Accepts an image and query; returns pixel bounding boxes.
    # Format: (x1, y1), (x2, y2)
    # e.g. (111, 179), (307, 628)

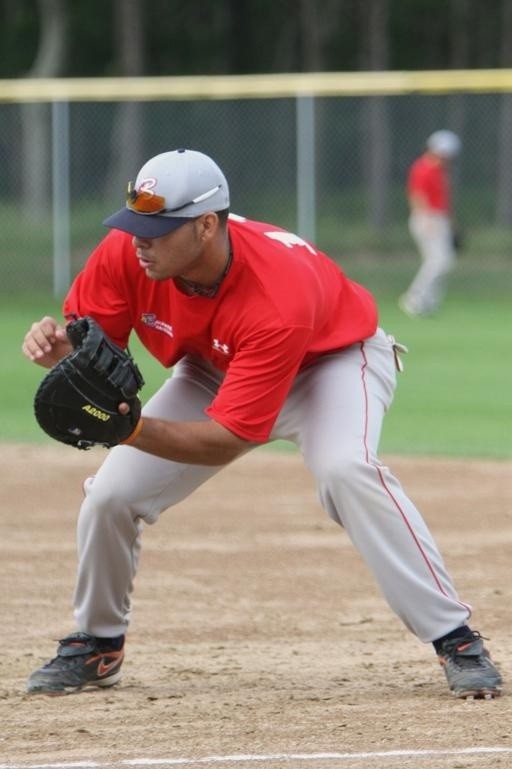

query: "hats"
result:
(100, 148), (229, 239)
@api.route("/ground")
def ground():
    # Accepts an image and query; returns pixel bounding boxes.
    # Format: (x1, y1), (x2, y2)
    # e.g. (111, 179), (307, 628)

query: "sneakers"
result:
(436, 628), (503, 698)
(22, 634), (125, 694)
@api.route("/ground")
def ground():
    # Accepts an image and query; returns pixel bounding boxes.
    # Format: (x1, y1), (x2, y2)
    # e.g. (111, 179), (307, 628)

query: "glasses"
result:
(125, 182), (222, 216)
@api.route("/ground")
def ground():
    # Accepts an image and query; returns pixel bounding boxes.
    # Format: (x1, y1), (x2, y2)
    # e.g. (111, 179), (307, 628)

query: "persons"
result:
(398, 129), (461, 315)
(20, 146), (505, 703)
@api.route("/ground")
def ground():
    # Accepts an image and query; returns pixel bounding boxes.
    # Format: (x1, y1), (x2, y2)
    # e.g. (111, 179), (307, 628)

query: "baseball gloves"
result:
(33, 317), (146, 450)
